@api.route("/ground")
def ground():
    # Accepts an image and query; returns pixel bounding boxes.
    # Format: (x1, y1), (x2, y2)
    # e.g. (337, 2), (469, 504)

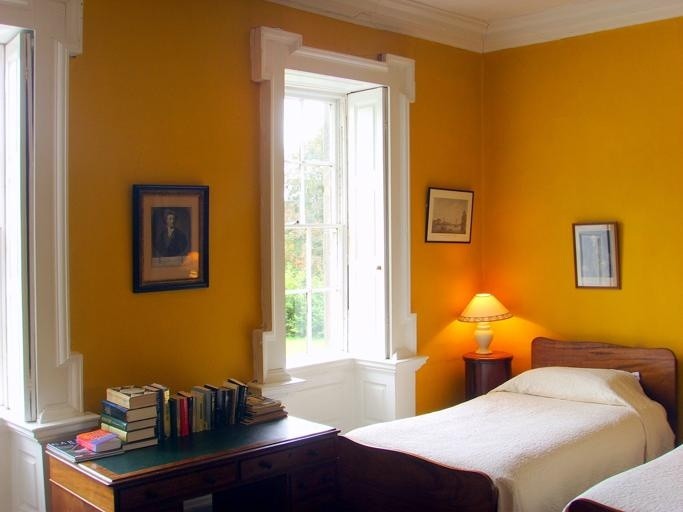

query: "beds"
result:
(340, 334), (678, 512)
(562, 444), (682, 511)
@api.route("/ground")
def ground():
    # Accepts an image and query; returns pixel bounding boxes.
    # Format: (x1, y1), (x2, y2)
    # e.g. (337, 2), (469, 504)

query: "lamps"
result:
(456, 291), (513, 354)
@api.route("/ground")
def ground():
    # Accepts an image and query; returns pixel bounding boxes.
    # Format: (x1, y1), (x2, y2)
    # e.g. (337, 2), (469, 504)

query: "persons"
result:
(154, 209), (189, 257)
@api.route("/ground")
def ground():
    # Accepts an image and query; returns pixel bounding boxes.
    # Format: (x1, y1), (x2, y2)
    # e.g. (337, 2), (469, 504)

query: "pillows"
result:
(490, 365), (641, 406)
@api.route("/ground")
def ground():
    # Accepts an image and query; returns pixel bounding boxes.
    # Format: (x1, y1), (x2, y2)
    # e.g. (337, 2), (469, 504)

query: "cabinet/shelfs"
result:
(44, 413), (340, 510)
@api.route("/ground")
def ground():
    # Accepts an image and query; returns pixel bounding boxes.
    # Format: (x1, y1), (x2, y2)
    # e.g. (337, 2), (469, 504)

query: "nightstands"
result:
(462, 353), (513, 401)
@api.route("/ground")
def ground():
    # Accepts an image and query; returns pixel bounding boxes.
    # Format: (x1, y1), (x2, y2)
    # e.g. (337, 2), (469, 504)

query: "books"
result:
(46, 378), (288, 463)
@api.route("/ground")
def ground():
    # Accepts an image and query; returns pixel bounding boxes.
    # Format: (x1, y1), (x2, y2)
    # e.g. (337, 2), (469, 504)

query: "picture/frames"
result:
(129, 180), (211, 294)
(424, 186), (475, 244)
(571, 221), (622, 291)
